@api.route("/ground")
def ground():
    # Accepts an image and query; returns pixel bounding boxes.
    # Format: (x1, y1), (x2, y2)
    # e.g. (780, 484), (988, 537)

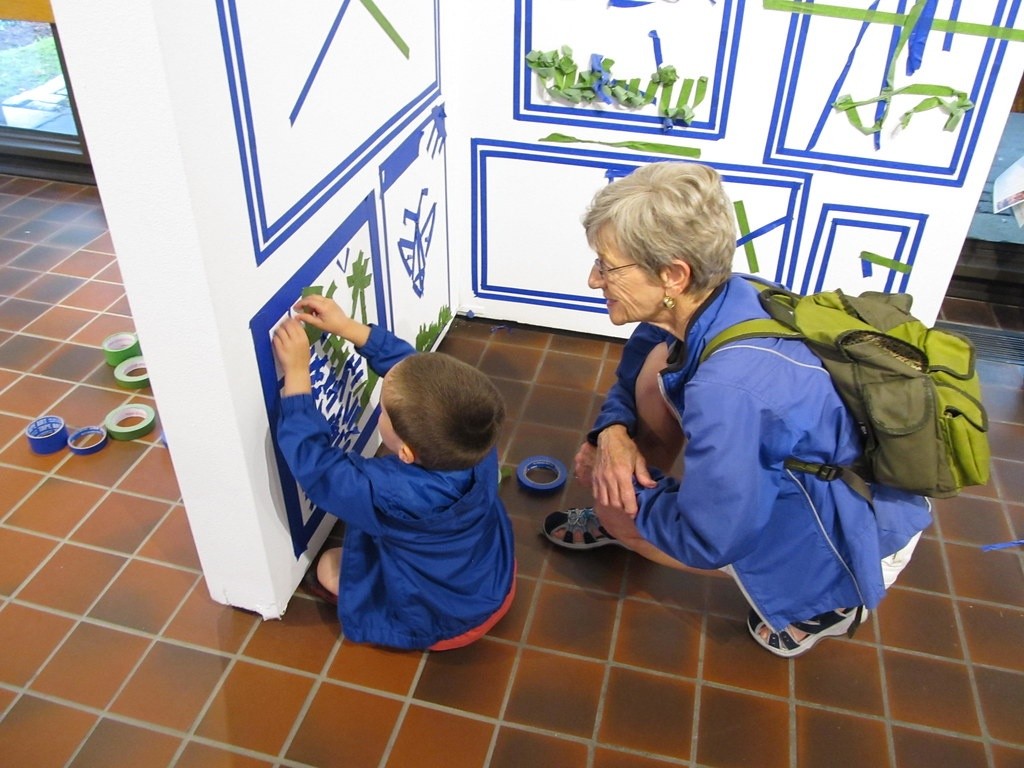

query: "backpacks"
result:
(699, 279), (991, 516)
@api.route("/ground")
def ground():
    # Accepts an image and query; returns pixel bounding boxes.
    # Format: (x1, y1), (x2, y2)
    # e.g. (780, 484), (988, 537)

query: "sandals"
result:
(543, 506), (634, 551)
(747, 605), (868, 658)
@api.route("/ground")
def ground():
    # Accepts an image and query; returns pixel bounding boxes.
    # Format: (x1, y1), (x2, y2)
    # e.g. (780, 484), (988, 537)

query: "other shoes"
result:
(306, 568), (338, 606)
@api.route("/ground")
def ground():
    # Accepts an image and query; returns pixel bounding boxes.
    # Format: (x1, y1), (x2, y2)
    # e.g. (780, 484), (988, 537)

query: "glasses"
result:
(595, 259), (637, 280)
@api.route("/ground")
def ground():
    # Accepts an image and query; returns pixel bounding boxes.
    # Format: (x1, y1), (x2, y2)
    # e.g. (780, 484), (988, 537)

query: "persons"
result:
(544, 160), (931, 658)
(272, 294), (519, 651)
